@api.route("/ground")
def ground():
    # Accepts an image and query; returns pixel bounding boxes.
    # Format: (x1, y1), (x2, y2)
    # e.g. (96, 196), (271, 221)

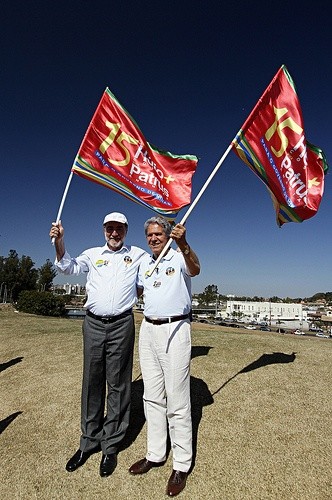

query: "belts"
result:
(86, 308), (132, 324)
(145, 314), (188, 325)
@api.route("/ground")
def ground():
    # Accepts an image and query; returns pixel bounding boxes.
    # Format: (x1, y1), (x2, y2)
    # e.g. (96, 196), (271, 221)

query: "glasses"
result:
(106, 226), (124, 233)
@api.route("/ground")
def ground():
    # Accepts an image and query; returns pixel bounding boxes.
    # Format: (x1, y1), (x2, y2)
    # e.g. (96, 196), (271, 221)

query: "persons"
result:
(49, 212), (150, 477)
(129, 215), (201, 496)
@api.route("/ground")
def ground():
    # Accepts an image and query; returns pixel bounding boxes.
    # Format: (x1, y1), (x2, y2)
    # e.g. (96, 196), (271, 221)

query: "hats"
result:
(102, 211), (129, 225)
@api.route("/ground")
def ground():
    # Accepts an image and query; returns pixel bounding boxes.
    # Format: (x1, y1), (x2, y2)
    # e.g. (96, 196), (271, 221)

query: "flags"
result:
(229, 64), (330, 230)
(71, 86), (200, 220)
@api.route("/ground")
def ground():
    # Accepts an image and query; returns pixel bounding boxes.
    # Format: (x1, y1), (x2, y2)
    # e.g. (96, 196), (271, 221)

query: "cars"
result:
(293, 330), (306, 335)
(309, 326), (323, 332)
(195, 315), (271, 332)
(316, 332), (330, 338)
(276, 327), (288, 334)
(243, 324), (257, 330)
(260, 326), (271, 332)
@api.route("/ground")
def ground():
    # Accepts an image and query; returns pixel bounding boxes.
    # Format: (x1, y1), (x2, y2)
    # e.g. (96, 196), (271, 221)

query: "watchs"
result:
(182, 246), (191, 256)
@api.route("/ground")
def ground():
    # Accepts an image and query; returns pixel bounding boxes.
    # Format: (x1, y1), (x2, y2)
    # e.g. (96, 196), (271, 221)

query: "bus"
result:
(191, 308), (221, 320)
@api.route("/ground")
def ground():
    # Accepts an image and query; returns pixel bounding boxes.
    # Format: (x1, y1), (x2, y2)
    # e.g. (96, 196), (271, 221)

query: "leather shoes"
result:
(65, 449), (90, 472)
(168, 469), (188, 496)
(100, 452), (117, 477)
(129, 456), (167, 474)
